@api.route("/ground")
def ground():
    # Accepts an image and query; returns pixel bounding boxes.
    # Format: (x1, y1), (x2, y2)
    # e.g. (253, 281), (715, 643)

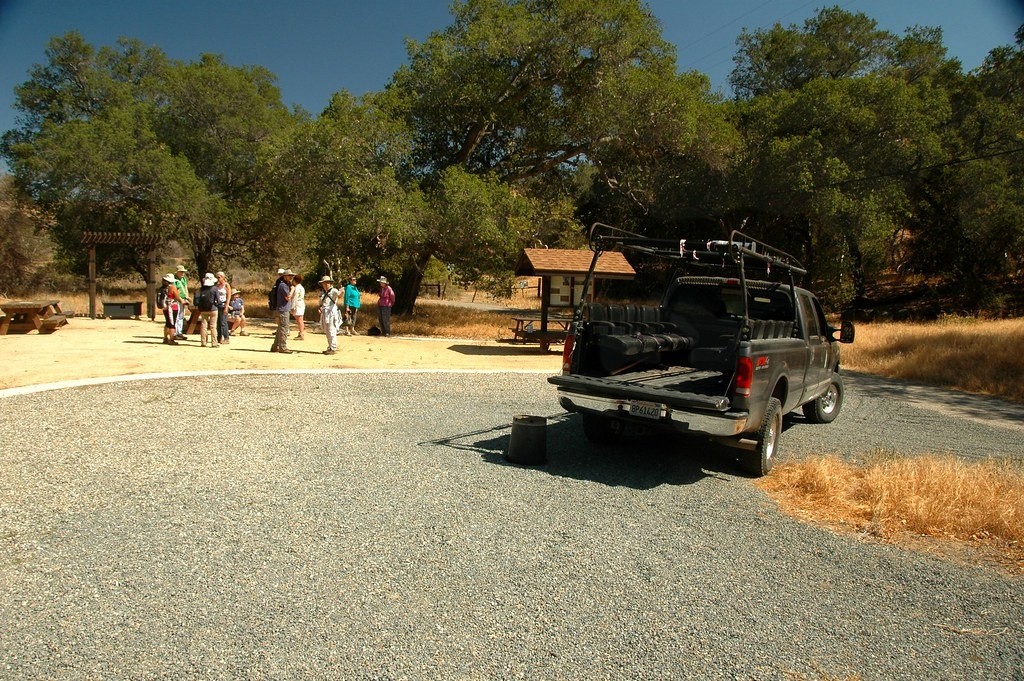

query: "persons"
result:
(377, 276), (395, 336)
(229, 289), (249, 336)
(344, 276), (361, 336)
(199, 271), (231, 347)
(318, 276), (345, 355)
(162, 264), (192, 345)
(271, 269), (295, 353)
(292, 275), (306, 340)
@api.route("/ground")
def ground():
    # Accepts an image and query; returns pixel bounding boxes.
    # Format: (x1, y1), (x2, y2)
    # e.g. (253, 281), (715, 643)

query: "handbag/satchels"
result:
(341, 311), (352, 326)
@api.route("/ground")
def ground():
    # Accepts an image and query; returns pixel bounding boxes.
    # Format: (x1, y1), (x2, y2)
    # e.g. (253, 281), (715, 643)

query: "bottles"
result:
(527, 324), (533, 333)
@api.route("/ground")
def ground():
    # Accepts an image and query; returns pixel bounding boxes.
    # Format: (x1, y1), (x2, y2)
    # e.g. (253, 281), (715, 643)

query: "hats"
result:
(202, 273), (218, 283)
(377, 276), (389, 284)
(162, 272), (178, 283)
(174, 265), (187, 273)
(231, 289), (241, 295)
(318, 276), (334, 285)
(277, 269), (285, 274)
(283, 270), (296, 276)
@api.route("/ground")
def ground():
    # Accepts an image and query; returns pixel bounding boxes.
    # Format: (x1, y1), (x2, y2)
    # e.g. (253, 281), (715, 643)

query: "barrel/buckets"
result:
(507, 414), (549, 465)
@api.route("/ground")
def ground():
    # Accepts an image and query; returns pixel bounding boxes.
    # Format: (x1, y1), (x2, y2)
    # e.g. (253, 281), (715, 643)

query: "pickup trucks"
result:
(553, 211), (850, 475)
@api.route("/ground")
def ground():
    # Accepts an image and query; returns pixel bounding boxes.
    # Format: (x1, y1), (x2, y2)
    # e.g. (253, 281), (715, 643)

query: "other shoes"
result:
(240, 331), (249, 336)
(345, 331), (352, 336)
(294, 336), (304, 340)
(201, 345), (205, 347)
(162, 334), (187, 345)
(218, 338), (223, 342)
(271, 348), (280, 351)
(385, 333), (390, 336)
(229, 330), (236, 335)
(220, 339), (229, 344)
(280, 348), (292, 353)
(351, 330), (358, 335)
(322, 349), (334, 355)
(213, 345), (219, 347)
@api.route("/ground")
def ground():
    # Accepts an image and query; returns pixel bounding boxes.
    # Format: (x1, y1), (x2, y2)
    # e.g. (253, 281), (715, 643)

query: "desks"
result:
(0, 300), (68, 335)
(183, 305), (234, 335)
(511, 317), (573, 345)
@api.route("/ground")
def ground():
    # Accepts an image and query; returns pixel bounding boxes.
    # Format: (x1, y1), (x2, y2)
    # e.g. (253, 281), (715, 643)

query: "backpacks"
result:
(157, 285), (170, 309)
(198, 286), (216, 312)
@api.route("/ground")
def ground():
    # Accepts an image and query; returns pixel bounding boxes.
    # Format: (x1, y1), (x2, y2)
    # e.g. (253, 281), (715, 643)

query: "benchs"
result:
(690, 319), (795, 370)
(43, 315), (66, 324)
(509, 328), (567, 339)
(187, 321), (211, 324)
(587, 303), (696, 355)
